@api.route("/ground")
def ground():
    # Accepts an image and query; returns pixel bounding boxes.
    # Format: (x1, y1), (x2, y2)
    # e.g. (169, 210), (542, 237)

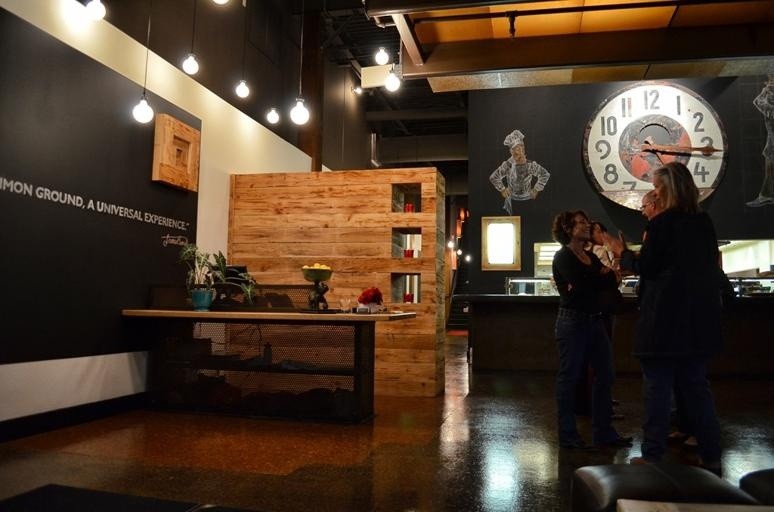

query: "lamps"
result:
(351, 85), (362, 95)
(384, 1), (401, 92)
(289, 2), (311, 125)
(181, 1), (199, 76)
(374, 1), (392, 68)
(84, 1), (107, 23)
(130, 2), (155, 125)
(234, 1), (250, 99)
(266, 1), (280, 124)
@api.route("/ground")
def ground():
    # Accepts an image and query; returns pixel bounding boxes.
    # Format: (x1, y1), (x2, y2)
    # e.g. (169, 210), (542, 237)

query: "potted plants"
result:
(178, 242), (258, 312)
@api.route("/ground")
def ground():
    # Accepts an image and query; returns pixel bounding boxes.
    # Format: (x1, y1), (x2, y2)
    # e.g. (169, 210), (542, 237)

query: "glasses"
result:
(640, 201), (652, 211)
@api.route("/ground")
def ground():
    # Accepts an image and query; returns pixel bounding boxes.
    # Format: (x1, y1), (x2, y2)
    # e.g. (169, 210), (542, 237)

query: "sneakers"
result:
(745, 192), (774, 207)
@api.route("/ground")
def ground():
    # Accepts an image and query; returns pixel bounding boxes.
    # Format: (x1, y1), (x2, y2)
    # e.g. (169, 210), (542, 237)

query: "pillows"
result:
(676, 467), (761, 503)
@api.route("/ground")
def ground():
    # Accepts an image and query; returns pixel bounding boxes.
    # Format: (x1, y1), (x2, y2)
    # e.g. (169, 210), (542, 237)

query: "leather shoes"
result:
(560, 439), (600, 452)
(610, 434), (634, 444)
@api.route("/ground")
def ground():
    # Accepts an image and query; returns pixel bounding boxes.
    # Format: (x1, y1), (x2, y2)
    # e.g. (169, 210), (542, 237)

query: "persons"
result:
(601, 162), (722, 467)
(552, 210), (633, 447)
(488, 130), (550, 209)
(745, 81), (774, 208)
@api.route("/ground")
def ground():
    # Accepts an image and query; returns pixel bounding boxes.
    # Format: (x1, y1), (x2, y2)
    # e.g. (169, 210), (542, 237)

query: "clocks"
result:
(583, 79), (729, 217)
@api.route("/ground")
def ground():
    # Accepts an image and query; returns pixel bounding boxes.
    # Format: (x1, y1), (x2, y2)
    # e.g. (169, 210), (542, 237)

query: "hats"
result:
(503, 130), (526, 149)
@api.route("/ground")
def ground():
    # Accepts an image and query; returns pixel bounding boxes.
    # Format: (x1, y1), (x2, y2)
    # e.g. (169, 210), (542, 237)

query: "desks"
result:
(121, 308), (417, 423)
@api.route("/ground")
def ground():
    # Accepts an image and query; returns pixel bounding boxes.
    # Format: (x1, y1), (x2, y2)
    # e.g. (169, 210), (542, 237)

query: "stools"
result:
(572, 459), (678, 512)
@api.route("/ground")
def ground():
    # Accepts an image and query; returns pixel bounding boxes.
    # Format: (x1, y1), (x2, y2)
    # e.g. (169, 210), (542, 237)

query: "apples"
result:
(303, 263), (332, 270)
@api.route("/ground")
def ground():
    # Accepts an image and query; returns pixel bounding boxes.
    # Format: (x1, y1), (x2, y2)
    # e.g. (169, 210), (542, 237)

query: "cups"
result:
(403, 293), (414, 303)
(404, 249), (414, 258)
(404, 201), (415, 212)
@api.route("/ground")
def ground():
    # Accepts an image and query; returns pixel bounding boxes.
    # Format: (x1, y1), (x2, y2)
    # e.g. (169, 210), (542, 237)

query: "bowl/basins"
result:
(301, 268), (332, 282)
